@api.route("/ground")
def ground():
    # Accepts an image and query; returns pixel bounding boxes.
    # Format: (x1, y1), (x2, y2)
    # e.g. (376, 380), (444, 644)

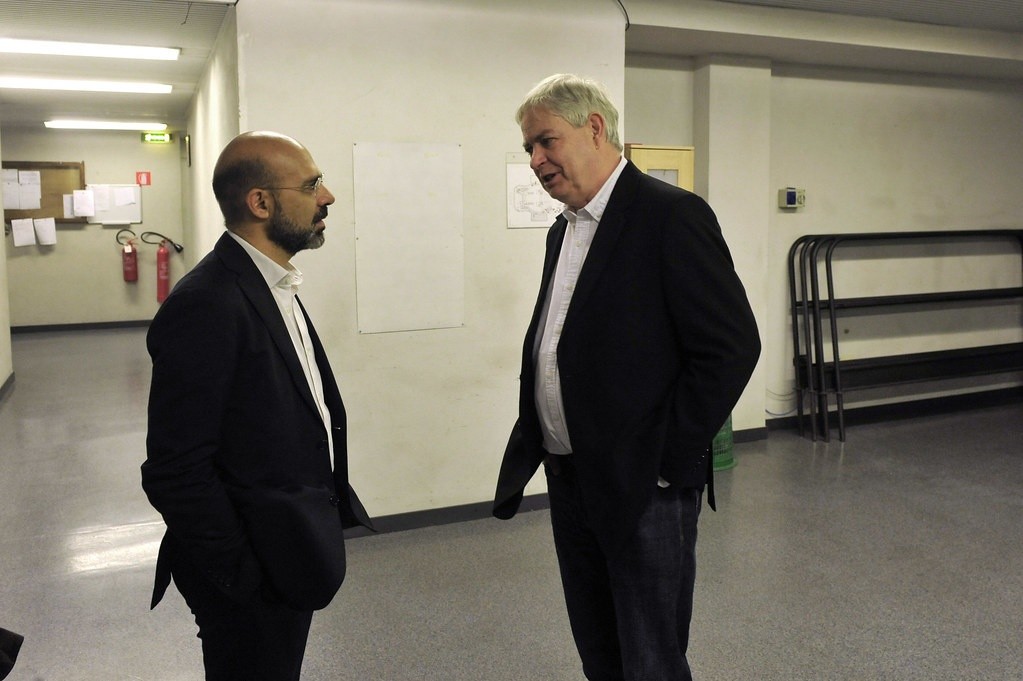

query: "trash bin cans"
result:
(712, 411), (737, 472)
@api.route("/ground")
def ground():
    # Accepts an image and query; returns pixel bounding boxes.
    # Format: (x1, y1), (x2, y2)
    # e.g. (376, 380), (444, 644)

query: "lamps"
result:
(0, 37), (181, 60)
(0, 74), (173, 95)
(44, 121), (168, 131)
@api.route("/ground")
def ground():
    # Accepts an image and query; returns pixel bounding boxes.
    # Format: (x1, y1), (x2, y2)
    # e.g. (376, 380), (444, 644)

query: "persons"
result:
(489, 72), (762, 681)
(140, 129), (377, 680)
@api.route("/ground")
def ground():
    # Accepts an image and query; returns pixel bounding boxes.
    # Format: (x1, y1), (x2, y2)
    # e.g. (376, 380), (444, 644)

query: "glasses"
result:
(255, 173), (322, 196)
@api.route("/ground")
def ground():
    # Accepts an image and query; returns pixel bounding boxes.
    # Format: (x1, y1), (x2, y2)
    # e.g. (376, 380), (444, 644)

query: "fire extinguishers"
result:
(115, 229), (139, 283)
(141, 231), (184, 304)
